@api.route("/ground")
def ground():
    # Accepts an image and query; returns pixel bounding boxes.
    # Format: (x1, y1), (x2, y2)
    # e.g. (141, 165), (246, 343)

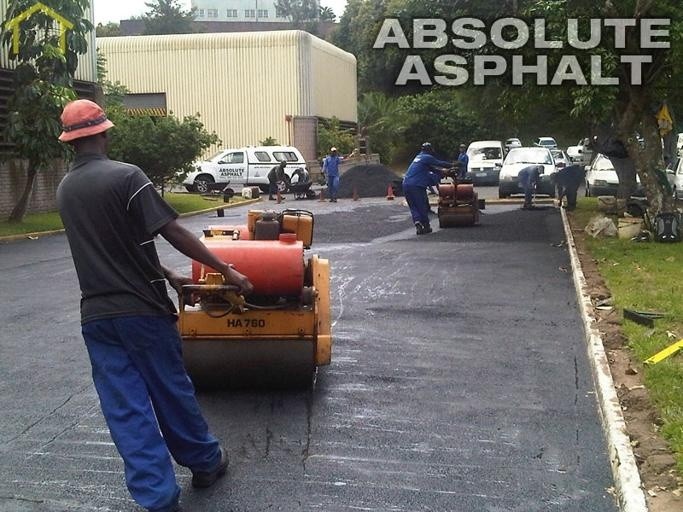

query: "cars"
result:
(465, 132), (683, 200)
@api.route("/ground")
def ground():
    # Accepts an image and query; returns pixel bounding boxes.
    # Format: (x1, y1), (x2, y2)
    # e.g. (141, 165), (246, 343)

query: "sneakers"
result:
(268, 195), (276, 201)
(412, 222), (434, 236)
(189, 444), (230, 488)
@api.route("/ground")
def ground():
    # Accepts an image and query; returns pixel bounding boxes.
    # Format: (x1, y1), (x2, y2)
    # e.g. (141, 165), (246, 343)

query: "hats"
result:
(330, 146), (336, 152)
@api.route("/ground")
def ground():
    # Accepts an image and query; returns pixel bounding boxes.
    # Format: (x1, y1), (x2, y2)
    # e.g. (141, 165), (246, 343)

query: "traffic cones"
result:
(317, 183), (395, 202)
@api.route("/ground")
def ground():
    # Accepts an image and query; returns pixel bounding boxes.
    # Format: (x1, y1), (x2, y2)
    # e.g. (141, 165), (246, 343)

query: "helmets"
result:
(54, 99), (116, 144)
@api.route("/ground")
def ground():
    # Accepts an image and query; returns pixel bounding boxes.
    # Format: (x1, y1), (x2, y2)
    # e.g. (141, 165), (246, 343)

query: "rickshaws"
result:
(284, 179), (313, 199)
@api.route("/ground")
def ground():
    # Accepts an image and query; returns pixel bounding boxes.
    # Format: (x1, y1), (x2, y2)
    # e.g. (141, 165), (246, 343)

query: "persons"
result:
(549, 164), (586, 211)
(320, 146), (338, 203)
(517, 164), (545, 210)
(400, 142), (464, 236)
(288, 167), (309, 199)
(266, 161), (286, 201)
(457, 144), (468, 180)
(53, 99), (256, 511)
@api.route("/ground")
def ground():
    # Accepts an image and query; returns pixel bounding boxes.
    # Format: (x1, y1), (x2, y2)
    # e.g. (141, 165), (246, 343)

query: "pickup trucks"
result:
(173, 147), (309, 194)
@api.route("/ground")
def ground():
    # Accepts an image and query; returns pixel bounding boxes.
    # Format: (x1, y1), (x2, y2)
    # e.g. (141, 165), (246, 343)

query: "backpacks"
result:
(652, 213), (679, 243)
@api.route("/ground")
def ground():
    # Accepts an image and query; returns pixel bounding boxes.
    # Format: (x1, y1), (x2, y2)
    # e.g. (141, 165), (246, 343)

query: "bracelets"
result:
(221, 263), (232, 284)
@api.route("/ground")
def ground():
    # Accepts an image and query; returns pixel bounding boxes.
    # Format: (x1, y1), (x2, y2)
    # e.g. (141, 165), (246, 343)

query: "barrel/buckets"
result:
(618, 219), (643, 239)
